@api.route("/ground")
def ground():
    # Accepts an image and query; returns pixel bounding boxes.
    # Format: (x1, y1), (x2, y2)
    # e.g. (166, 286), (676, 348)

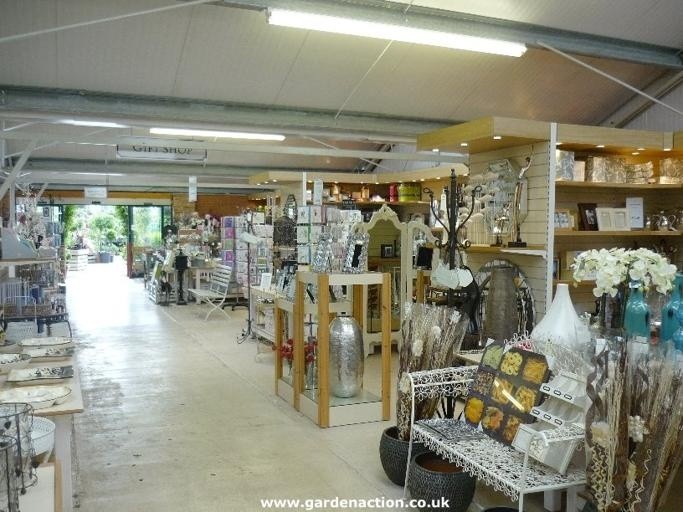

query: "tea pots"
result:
(651, 210), (676, 231)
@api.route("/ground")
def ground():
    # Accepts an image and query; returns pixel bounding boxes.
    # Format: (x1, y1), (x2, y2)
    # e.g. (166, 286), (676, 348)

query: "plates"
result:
(0, 352), (31, 371)
(17, 337), (73, 348)
(21, 346), (75, 361)
(8, 365), (75, 385)
(4, 386), (72, 408)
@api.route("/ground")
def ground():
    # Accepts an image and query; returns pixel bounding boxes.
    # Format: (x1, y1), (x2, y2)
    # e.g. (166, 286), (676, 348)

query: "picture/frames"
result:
(555, 203), (598, 231)
(381, 243), (393, 258)
(553, 257), (561, 280)
(596, 207), (631, 230)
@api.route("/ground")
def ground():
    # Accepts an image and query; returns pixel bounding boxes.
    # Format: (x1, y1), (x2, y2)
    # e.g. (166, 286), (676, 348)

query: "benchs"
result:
(186, 263), (232, 321)
(401, 363), (587, 512)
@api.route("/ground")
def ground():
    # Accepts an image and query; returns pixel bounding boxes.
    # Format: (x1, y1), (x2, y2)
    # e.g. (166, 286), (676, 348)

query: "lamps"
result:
(147, 127), (287, 141)
(261, 7), (528, 60)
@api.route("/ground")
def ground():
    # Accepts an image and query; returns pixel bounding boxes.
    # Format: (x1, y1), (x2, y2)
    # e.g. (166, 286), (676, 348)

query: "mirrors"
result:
(362, 204), (408, 359)
(408, 220), (439, 304)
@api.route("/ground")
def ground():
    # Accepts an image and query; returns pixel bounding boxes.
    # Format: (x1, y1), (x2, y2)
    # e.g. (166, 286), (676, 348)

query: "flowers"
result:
(575, 248), (677, 326)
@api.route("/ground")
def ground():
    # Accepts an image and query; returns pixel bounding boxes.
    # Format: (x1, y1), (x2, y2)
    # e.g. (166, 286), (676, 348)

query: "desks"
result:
(0, 338), (85, 512)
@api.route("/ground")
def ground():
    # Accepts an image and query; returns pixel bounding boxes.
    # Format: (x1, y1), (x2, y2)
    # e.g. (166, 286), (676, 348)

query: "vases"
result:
(530, 283), (591, 350)
(660, 272), (683, 340)
(408, 451), (476, 512)
(626, 280), (651, 339)
(379, 426), (429, 487)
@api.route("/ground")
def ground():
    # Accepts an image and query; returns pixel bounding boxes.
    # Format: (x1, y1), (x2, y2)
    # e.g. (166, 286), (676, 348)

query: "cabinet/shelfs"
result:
(250, 280), (304, 345)
(307, 179), (433, 223)
(553, 141), (683, 315)
(0, 223), (68, 320)
(274, 265), (392, 429)
(248, 192), (279, 223)
(144, 246), (184, 303)
